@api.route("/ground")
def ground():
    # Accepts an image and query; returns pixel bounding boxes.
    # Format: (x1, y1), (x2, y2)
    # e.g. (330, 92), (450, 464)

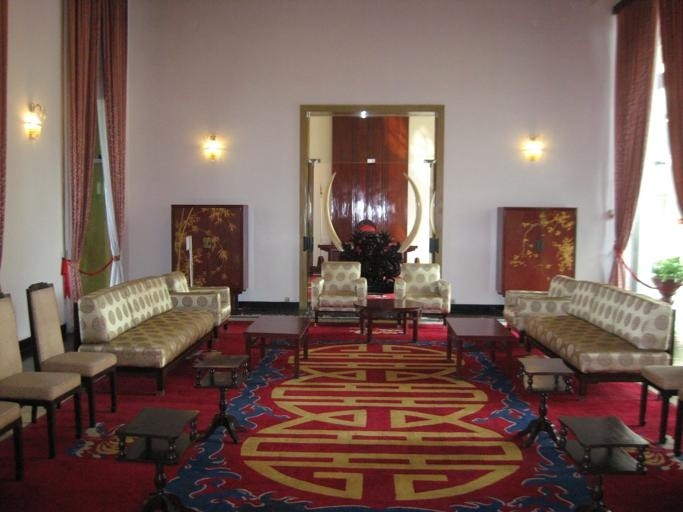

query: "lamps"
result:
(522, 136), (544, 159)
(204, 134), (224, 161)
(25, 103), (43, 138)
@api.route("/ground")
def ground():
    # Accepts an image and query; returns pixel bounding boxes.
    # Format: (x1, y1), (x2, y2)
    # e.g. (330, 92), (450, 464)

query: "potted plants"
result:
(652, 257), (683, 304)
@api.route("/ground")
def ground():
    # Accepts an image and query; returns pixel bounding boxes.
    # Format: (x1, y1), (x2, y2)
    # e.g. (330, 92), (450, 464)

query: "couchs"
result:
(78, 276), (215, 395)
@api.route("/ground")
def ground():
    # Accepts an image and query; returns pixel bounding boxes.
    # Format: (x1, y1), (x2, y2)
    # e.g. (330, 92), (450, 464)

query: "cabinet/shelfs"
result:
(497, 207), (577, 296)
(171, 204), (248, 305)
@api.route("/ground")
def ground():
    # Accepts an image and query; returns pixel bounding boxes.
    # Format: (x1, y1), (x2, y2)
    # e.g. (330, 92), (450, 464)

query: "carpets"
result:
(1, 320), (682, 511)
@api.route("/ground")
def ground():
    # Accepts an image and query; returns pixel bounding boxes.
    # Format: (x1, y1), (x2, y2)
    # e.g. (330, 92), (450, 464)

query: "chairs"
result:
(165, 271), (231, 339)
(640, 365), (683, 443)
(0, 402), (23, 480)
(26, 282), (117, 427)
(394, 263), (450, 326)
(504, 275), (576, 345)
(311, 262), (367, 323)
(525, 281), (676, 397)
(1, 294), (81, 457)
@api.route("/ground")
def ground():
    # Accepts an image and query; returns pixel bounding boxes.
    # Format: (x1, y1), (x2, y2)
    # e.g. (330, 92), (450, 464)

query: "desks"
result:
(446, 318), (515, 376)
(353, 299), (422, 341)
(243, 317), (308, 378)
